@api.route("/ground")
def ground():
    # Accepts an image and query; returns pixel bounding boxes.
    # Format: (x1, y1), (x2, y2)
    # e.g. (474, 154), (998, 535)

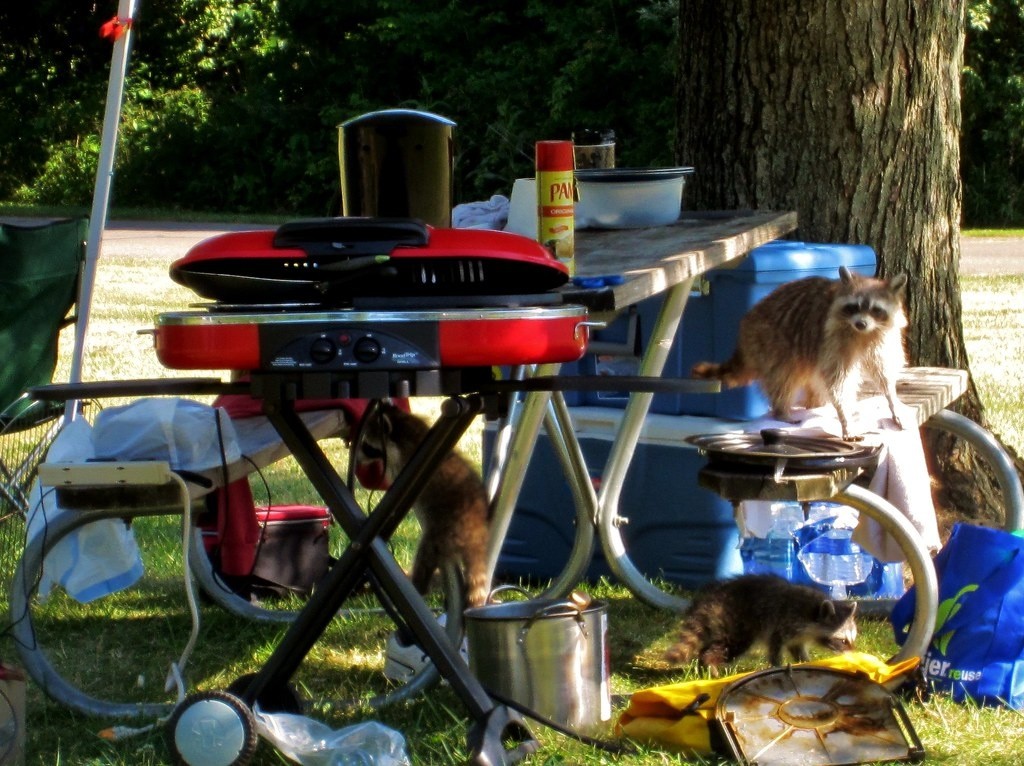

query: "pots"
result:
(462, 584), (611, 739)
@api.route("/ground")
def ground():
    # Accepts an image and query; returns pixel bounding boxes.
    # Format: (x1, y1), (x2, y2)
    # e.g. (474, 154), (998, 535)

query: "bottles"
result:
(744, 502), (903, 601)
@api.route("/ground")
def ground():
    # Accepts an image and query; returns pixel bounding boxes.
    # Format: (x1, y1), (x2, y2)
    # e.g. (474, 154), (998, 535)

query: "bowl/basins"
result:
(575, 177), (685, 229)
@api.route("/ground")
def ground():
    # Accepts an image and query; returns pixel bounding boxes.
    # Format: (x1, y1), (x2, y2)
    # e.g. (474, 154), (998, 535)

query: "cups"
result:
(571, 126), (616, 202)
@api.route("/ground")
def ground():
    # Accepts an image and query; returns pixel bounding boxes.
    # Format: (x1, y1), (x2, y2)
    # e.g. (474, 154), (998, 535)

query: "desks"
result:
(234, 364), (495, 726)
(470, 210), (800, 617)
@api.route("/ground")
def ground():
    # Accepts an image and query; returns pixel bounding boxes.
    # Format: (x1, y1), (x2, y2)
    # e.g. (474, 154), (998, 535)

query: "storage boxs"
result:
(202, 505), (331, 601)
(497, 240), (877, 423)
(482, 403), (792, 592)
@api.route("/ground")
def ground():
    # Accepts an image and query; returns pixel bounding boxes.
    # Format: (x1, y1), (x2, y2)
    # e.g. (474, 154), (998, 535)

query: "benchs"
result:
(11, 404), (344, 722)
(698, 366), (1023, 694)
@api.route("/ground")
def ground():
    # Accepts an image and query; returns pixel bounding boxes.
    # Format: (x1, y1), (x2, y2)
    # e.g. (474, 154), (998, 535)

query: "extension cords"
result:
(38, 461), (171, 484)
